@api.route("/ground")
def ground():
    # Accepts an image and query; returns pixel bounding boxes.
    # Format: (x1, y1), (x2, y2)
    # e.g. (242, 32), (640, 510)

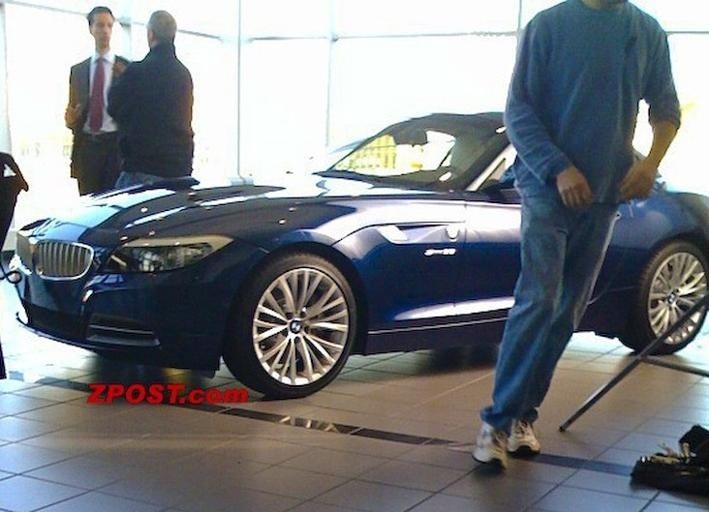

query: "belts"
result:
(80, 131), (116, 144)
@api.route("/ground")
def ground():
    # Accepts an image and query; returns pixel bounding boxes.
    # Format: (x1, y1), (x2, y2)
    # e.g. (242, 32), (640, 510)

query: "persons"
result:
(469, 0), (681, 473)
(107, 8), (195, 188)
(62, 4), (133, 196)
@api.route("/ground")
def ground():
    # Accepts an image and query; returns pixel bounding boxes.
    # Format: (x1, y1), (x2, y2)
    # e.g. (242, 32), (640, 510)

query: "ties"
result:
(88, 58), (105, 133)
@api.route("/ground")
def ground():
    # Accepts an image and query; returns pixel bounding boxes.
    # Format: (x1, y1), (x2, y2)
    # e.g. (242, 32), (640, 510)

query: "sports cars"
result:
(12, 111), (708, 399)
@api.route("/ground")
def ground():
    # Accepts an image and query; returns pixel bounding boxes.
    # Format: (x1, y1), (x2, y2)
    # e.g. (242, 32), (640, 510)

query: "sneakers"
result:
(472, 419), (509, 471)
(508, 417), (540, 457)
